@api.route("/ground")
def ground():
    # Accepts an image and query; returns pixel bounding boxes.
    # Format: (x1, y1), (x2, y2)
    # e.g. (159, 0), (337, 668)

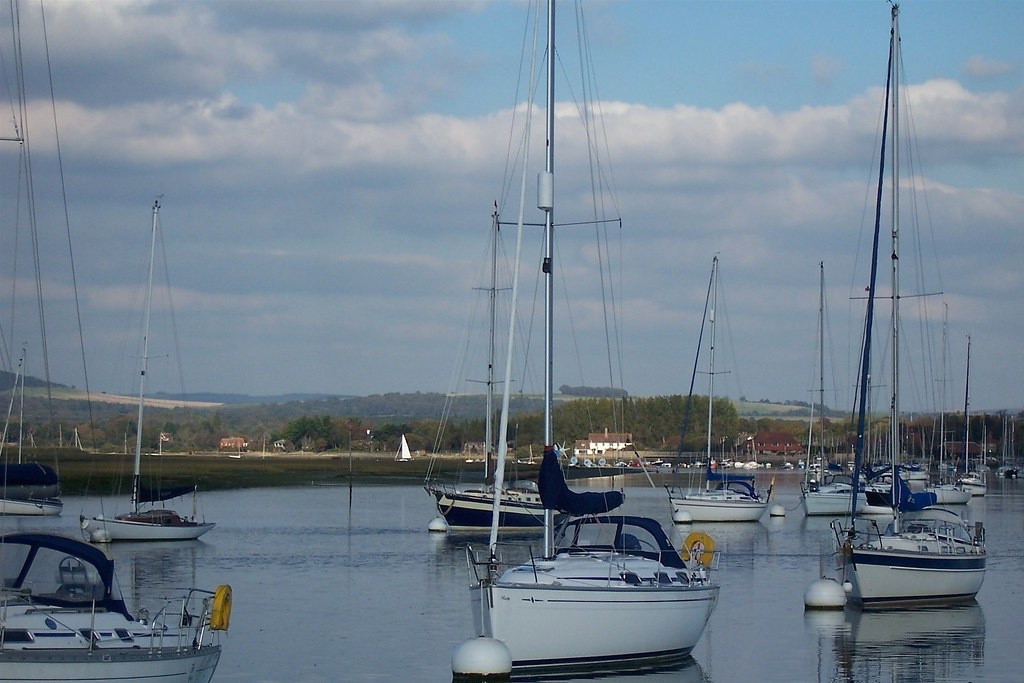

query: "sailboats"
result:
(394, 433), (412, 463)
(421, 0), (1024, 683)
(0, 1), (232, 680)
